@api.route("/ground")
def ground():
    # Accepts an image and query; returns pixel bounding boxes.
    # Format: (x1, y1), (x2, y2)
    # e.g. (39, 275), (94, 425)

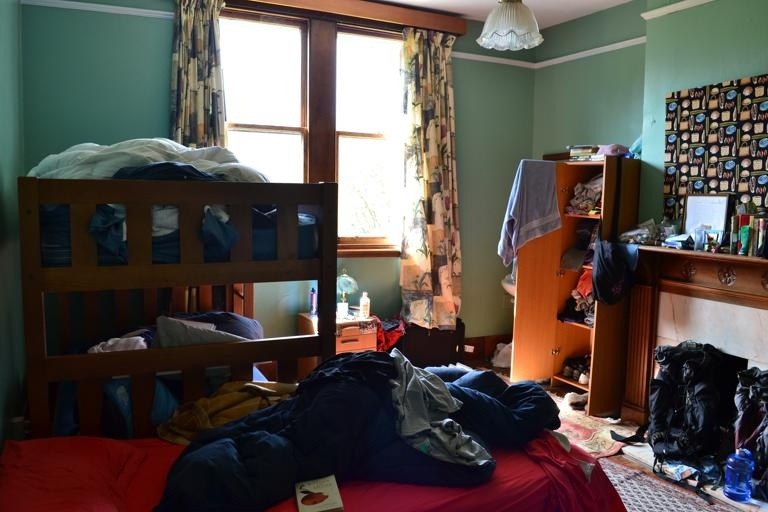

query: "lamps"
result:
(476, 0), (544, 51)
(337, 268), (359, 303)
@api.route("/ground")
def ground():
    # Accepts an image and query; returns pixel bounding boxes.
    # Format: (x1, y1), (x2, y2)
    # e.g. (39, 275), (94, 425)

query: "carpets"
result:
(595, 457), (739, 512)
(478, 366), (636, 460)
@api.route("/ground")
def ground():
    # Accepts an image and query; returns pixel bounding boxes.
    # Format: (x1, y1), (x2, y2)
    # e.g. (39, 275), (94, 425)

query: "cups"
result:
(337, 303), (348, 319)
(694, 229), (705, 250)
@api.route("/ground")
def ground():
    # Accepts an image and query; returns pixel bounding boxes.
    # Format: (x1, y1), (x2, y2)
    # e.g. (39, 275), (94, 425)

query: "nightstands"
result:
(297, 312), (378, 377)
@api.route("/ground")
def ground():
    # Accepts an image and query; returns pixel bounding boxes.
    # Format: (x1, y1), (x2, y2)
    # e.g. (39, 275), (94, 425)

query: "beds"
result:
(16, 176), (338, 440)
(0, 437), (630, 512)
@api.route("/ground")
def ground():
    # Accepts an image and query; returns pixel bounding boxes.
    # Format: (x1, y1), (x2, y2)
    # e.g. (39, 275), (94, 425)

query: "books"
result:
(730, 214), (768, 258)
(295, 474), (344, 512)
(569, 145), (604, 162)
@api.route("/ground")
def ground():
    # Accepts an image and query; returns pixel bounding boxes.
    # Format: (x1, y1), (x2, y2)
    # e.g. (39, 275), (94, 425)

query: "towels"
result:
(497, 159), (563, 280)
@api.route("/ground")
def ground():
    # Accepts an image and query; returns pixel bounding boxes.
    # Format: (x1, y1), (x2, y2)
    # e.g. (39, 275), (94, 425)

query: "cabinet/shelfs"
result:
(509, 154), (641, 415)
(616, 238), (768, 427)
(394, 315), (465, 369)
